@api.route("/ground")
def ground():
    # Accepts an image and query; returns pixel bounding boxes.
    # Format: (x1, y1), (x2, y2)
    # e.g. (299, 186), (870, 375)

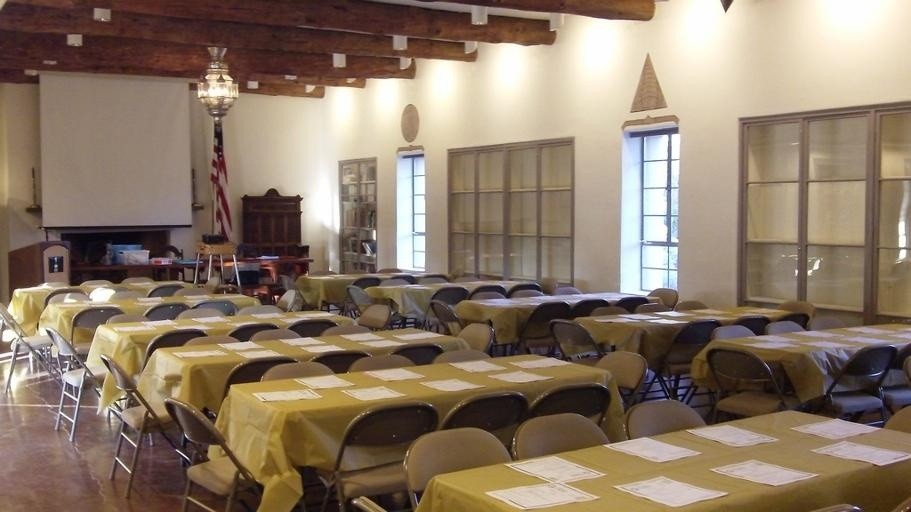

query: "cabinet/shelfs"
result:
(242, 190), (310, 257)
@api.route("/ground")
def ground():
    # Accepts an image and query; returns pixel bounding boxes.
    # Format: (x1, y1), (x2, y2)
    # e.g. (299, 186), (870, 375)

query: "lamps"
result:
(247, 5), (564, 94)
(26, 9), (110, 77)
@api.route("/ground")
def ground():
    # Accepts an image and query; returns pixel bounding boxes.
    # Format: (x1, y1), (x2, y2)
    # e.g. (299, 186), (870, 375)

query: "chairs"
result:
(0, 266), (911, 512)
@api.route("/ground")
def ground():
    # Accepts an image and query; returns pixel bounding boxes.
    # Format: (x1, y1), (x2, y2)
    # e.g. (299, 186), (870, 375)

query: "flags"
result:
(210, 120), (234, 240)
